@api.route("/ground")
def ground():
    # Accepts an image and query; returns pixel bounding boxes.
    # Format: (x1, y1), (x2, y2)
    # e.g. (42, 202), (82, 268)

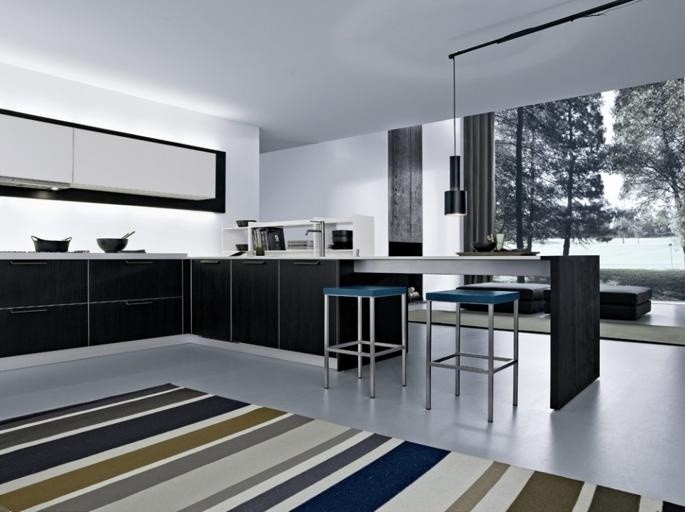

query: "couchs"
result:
(457, 283), (655, 321)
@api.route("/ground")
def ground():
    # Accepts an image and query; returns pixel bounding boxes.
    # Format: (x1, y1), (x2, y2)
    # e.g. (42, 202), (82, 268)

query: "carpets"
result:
(407, 308), (685, 348)
(0, 384), (684, 511)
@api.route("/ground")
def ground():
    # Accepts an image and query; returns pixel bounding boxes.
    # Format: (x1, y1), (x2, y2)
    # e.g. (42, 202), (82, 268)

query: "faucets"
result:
(305, 220), (325, 257)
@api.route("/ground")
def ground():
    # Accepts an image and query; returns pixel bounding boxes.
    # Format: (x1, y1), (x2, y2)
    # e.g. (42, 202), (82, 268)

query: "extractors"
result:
(0, 175), (69, 194)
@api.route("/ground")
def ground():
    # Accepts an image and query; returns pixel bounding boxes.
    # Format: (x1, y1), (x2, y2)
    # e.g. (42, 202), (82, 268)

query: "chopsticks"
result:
(121, 231), (136, 239)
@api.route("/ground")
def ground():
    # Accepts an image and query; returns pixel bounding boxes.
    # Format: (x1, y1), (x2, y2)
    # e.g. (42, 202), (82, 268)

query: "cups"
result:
(496, 232), (505, 251)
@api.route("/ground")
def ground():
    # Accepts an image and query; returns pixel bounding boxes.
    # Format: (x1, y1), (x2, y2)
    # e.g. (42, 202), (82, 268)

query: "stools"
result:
(320, 284), (408, 400)
(425, 288), (521, 424)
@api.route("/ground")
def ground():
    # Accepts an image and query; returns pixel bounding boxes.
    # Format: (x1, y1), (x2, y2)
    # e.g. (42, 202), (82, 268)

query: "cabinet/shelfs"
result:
(231, 259), (280, 358)
(0, 260), (87, 371)
(0, 114), (73, 191)
(87, 259), (189, 358)
(189, 257), (232, 350)
(222, 215), (373, 261)
(281, 258), (337, 371)
(73, 125), (219, 202)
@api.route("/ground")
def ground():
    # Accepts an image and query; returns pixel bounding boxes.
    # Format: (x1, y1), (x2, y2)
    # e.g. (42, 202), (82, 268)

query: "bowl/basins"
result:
(330, 229), (354, 249)
(95, 237), (128, 253)
(472, 241), (496, 252)
(234, 243), (248, 250)
(234, 219), (257, 227)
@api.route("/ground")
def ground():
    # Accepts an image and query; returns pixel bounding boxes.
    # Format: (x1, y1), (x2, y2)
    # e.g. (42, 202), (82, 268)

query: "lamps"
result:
(443, 54), (470, 217)
